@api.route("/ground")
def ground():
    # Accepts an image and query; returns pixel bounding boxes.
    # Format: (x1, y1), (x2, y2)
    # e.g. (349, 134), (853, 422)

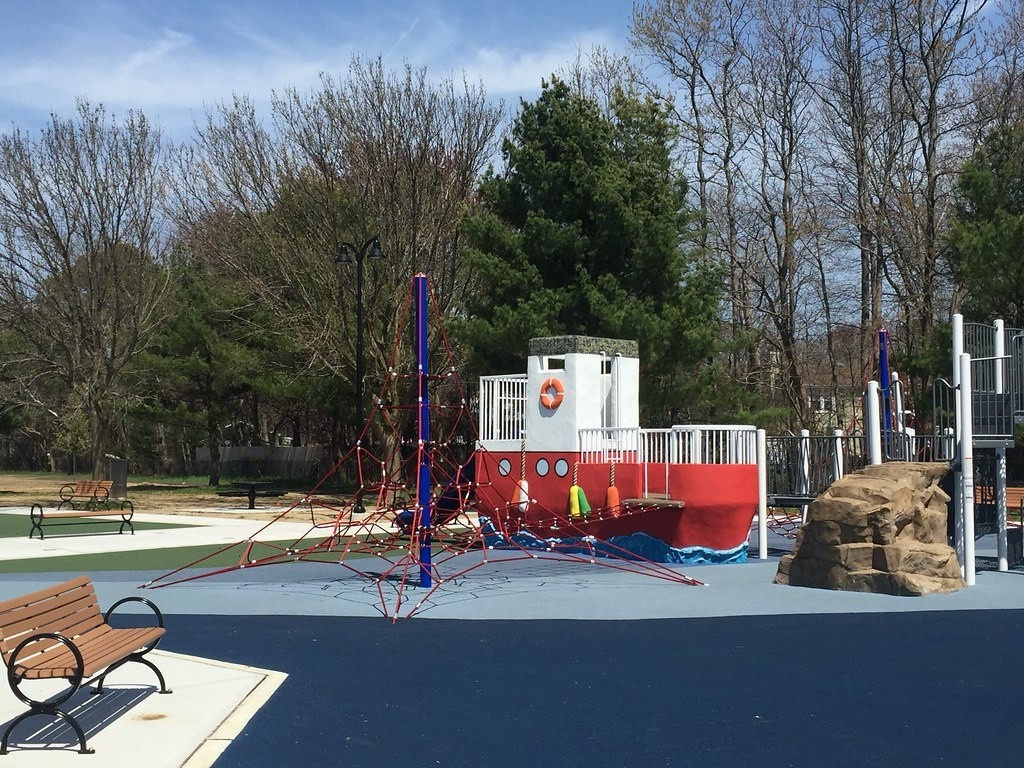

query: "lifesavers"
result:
(539, 376), (565, 410)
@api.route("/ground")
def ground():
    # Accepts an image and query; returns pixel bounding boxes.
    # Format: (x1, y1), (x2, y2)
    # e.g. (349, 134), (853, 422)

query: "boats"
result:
(477, 335), (762, 566)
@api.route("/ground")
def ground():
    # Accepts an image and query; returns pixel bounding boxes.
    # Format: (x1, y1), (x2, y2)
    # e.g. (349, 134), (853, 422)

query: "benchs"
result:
(216, 490), (290, 498)
(56, 478), (114, 511)
(975, 484), (1024, 526)
(0, 574), (174, 755)
(29, 500), (135, 541)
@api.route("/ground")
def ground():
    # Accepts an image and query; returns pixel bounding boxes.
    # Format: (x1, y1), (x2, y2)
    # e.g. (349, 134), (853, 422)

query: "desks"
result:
(231, 479), (273, 509)
